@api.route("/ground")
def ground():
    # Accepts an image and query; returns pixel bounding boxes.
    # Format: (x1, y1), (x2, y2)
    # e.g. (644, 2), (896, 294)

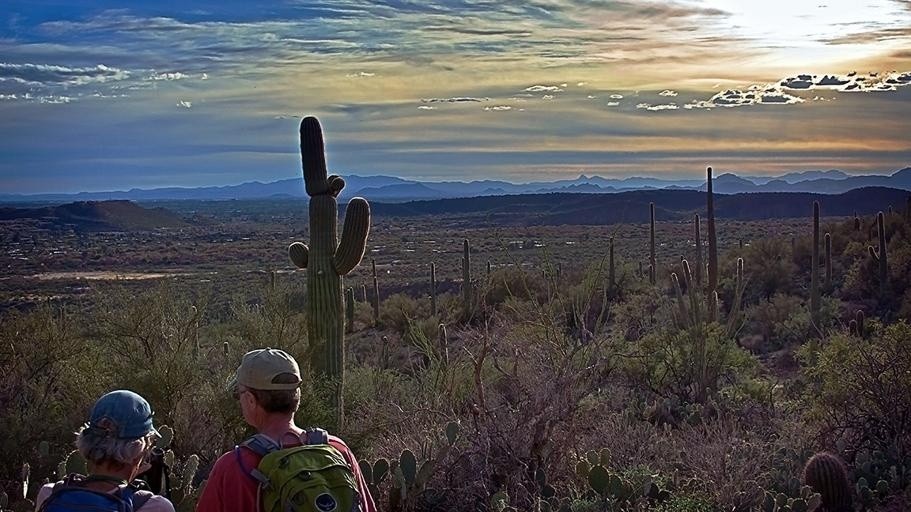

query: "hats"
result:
(88, 390), (164, 442)
(235, 346), (304, 392)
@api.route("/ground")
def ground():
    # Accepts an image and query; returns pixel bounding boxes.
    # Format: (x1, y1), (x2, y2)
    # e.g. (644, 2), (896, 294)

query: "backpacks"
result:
(243, 427), (362, 512)
(34, 474), (149, 512)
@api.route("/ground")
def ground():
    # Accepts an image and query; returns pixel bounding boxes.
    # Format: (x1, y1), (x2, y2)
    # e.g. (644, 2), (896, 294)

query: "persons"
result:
(35, 390), (175, 512)
(196, 347), (378, 512)
